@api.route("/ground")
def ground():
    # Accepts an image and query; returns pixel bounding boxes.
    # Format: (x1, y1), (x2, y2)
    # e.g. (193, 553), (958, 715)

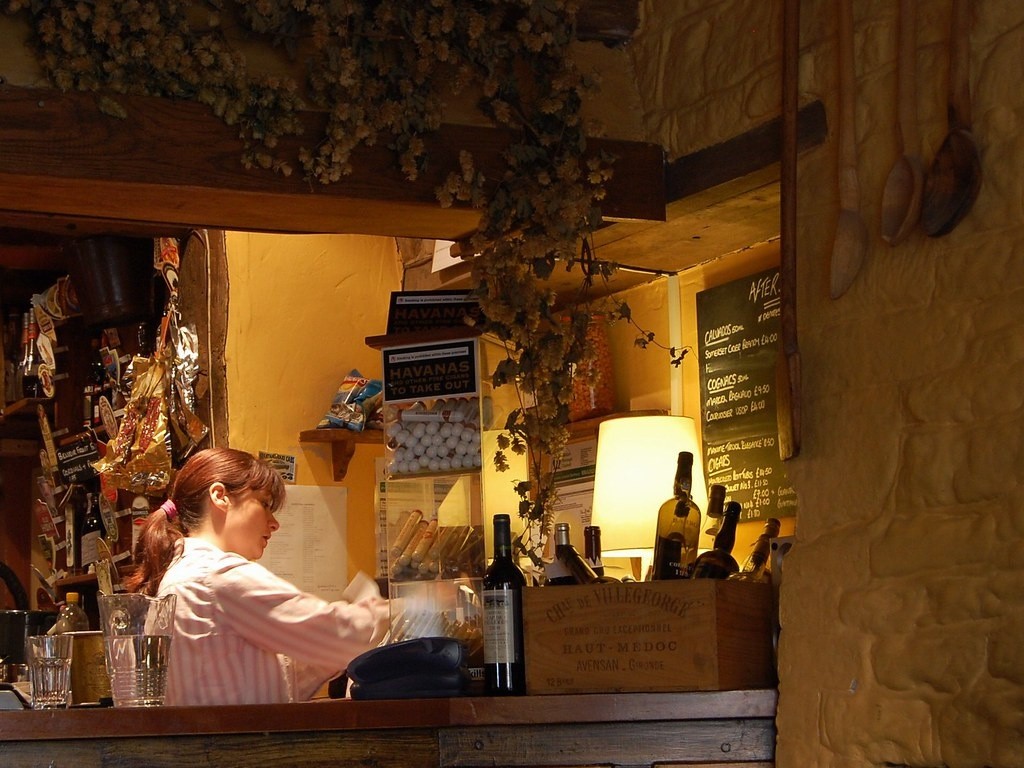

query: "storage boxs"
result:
(525, 579), (776, 695)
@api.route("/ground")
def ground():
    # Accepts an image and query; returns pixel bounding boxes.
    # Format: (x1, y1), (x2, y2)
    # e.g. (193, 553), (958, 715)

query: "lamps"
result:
(583, 415), (720, 578)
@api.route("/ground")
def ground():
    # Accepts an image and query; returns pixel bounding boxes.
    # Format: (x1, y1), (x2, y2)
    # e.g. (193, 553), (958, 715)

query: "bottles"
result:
(697, 484), (727, 560)
(544, 523), (577, 585)
(690, 501), (741, 580)
(652, 451), (702, 580)
(559, 544), (622, 585)
(739, 517), (781, 581)
(483, 513), (527, 697)
(16, 312), (30, 400)
(22, 307), (46, 398)
(584, 526), (604, 577)
(56, 593), (89, 636)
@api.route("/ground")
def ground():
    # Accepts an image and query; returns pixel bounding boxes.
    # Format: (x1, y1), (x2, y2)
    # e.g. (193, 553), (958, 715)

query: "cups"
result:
(28, 635), (74, 710)
(97, 592), (177, 708)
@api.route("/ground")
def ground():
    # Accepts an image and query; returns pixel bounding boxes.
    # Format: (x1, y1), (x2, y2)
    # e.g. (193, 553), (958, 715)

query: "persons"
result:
(126, 448), (399, 706)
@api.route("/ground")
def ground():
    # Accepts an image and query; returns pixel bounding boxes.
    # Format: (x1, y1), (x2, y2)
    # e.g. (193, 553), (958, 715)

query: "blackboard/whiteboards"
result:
(695, 265), (796, 523)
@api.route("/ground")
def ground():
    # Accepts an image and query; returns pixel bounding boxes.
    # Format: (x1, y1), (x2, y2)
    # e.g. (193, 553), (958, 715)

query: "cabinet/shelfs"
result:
(363, 328), (533, 695)
(58, 333), (146, 586)
